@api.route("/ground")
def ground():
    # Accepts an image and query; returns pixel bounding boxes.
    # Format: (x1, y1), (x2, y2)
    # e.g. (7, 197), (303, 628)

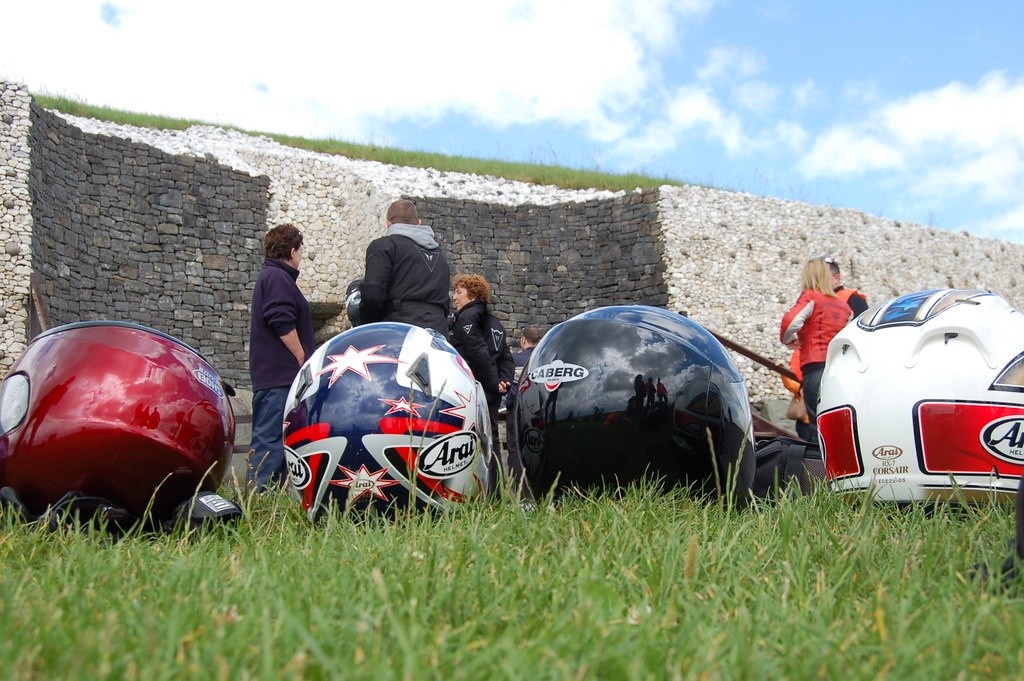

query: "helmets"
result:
(514, 304), (754, 510)
(1, 321), (236, 527)
(818, 290), (1024, 510)
(282, 322), (492, 526)
(345, 282), (362, 326)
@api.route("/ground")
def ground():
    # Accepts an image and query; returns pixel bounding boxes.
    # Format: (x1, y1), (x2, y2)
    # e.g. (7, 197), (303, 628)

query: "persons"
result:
(545, 383), (563, 419)
(249, 223), (315, 493)
(780, 255), (867, 446)
(634, 374), (669, 407)
(13, 377), (76, 453)
(346, 198), (539, 505)
(131, 403), (161, 430)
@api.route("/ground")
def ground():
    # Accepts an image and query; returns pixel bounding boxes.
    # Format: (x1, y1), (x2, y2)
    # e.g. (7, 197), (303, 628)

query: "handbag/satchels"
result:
(787, 394), (807, 420)
(750, 438), (827, 512)
